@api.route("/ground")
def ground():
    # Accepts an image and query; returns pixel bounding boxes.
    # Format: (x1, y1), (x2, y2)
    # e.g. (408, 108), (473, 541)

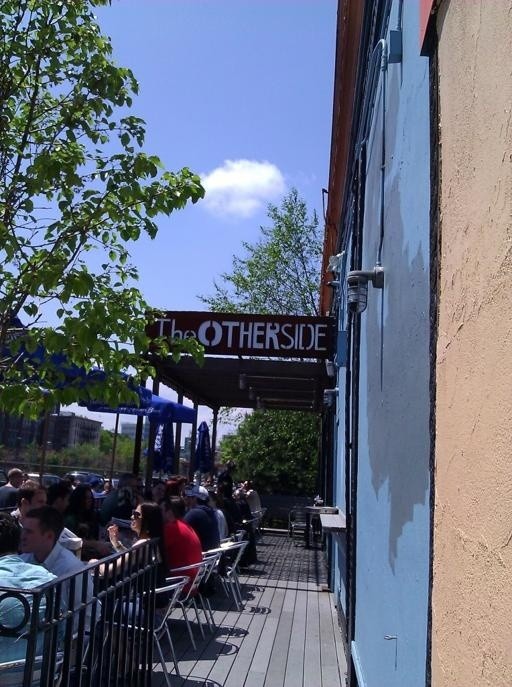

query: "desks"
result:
(304, 506), (339, 550)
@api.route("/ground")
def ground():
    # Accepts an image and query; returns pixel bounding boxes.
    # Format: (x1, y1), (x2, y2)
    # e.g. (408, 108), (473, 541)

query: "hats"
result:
(185, 486), (208, 500)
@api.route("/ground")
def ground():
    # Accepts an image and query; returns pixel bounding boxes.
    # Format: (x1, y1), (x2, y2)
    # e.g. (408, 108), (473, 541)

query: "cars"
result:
(66, 469), (106, 492)
(0, 470), (10, 488)
(105, 477), (120, 489)
(25, 471), (76, 492)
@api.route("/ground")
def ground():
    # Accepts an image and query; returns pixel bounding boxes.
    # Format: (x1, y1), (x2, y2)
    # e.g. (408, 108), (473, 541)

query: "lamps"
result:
(347, 265), (385, 314)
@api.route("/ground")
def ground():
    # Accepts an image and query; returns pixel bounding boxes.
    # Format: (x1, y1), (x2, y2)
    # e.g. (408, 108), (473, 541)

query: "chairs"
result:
(1, 575), (189, 687)
(219, 508), (268, 600)
(287, 508), (314, 545)
(151, 540), (250, 652)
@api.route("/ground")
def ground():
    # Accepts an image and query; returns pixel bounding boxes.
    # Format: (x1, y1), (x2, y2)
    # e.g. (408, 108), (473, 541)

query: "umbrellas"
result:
(1, 310), (213, 486)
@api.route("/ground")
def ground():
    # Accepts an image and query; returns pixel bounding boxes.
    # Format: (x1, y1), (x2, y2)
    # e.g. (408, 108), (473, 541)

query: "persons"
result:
(0, 510), (71, 686)
(1, 465), (262, 636)
